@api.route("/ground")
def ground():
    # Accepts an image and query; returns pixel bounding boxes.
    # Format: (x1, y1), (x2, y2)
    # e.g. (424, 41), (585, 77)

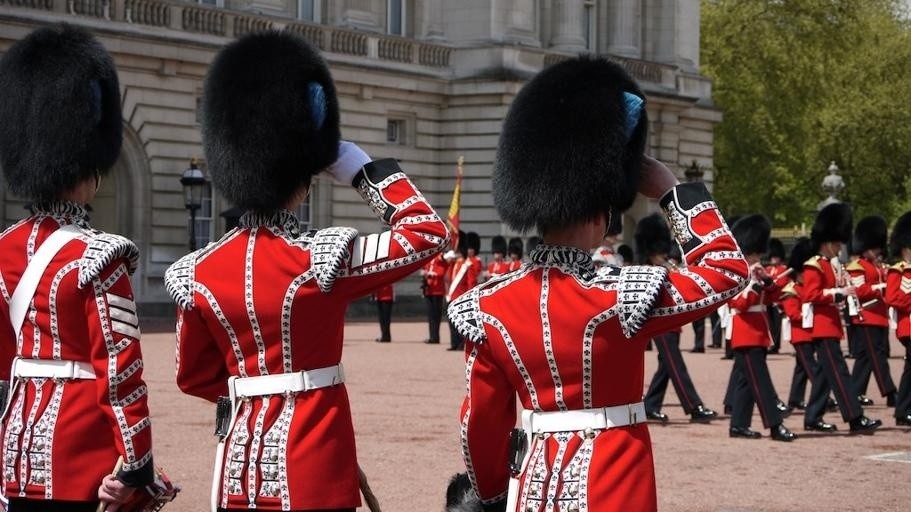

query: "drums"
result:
(109, 460), (181, 511)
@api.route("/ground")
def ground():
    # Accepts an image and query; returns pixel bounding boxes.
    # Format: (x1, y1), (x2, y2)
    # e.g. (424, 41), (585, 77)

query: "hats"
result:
(811, 203), (852, 251)
(201, 30), (338, 214)
(0, 21), (122, 199)
(617, 245), (632, 265)
(724, 213), (814, 276)
(491, 52), (648, 235)
(634, 211), (670, 257)
(853, 215), (887, 255)
(890, 210), (911, 258)
(458, 230), (542, 259)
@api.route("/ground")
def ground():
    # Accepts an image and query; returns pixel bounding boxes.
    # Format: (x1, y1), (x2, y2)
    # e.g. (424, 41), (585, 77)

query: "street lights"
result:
(685, 159), (703, 187)
(178, 154), (204, 254)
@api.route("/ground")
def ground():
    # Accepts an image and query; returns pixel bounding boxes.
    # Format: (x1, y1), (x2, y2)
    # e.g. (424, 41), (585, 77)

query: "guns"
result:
(484, 255), (488, 281)
(420, 265), (429, 298)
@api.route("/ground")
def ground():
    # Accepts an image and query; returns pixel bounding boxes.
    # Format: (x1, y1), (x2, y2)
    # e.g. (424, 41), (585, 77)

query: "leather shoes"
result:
(724, 404), (731, 415)
(650, 411), (667, 420)
(447, 348), (462, 350)
(771, 391), (909, 442)
(691, 405), (717, 421)
(691, 344), (732, 360)
(424, 340), (441, 344)
(729, 426), (761, 439)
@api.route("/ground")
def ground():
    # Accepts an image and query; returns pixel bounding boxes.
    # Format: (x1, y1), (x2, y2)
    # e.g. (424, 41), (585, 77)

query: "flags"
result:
(443, 158), (465, 252)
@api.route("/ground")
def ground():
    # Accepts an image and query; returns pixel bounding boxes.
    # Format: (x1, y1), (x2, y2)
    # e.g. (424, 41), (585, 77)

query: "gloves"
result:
(325, 139), (372, 186)
(442, 250), (454, 260)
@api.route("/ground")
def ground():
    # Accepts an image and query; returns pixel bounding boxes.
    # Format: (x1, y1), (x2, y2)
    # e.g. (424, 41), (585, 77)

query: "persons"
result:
(164, 31), (448, 512)
(0, 21), (155, 511)
(364, 202), (910, 440)
(445, 51), (749, 512)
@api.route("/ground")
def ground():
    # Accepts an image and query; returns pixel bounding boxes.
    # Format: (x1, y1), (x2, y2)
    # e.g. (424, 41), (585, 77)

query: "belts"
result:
(14, 359), (97, 380)
(227, 363), (345, 402)
(522, 401), (647, 434)
(730, 304), (767, 315)
